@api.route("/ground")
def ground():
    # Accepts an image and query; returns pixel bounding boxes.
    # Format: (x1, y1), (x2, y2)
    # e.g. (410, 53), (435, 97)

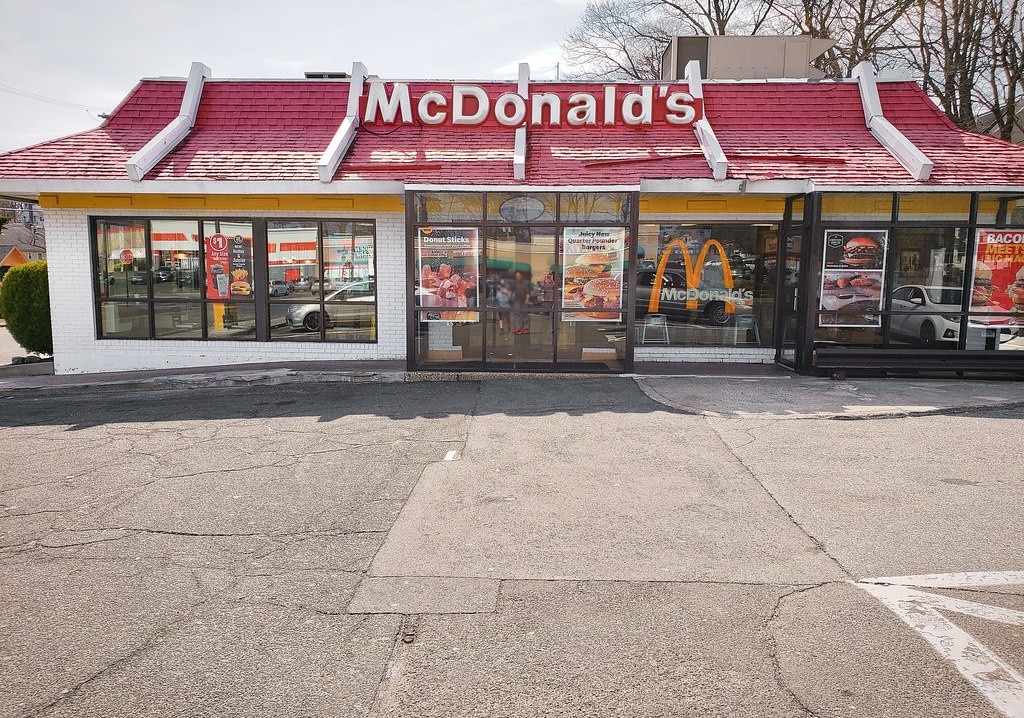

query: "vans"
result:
(269, 280), (290, 296)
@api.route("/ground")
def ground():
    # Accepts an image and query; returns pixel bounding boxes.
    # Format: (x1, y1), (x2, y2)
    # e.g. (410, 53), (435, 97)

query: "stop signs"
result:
(119, 250), (132, 265)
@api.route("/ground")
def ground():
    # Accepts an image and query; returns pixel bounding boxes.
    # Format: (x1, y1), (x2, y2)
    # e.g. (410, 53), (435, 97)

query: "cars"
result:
(294, 277), (311, 292)
(875, 284), (1019, 347)
(700, 250), (801, 287)
(311, 279), (333, 295)
(285, 280), (441, 332)
(99, 273), (115, 285)
(129, 272), (156, 285)
(155, 266), (208, 287)
(614, 267), (753, 326)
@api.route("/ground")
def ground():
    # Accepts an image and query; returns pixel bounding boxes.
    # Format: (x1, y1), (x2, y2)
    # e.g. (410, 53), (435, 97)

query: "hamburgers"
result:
(972, 260), (993, 303)
(563, 254), (621, 319)
(231, 281), (250, 296)
(843, 237), (877, 264)
(1008, 265), (1024, 313)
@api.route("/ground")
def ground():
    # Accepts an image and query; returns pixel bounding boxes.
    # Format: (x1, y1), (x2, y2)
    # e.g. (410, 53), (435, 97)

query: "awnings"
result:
(548, 261), (656, 273)
(415, 256), (531, 272)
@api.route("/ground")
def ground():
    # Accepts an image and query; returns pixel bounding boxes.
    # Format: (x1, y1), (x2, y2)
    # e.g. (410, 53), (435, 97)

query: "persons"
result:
(496, 272), (531, 334)
(541, 273), (555, 314)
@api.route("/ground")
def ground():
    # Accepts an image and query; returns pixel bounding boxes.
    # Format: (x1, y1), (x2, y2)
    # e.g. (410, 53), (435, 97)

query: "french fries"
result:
(232, 268), (248, 280)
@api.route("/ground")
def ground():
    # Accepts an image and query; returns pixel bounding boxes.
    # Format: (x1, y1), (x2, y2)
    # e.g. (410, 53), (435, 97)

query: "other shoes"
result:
(514, 327), (521, 334)
(508, 327), (512, 334)
(523, 328), (530, 335)
(500, 328), (504, 335)
